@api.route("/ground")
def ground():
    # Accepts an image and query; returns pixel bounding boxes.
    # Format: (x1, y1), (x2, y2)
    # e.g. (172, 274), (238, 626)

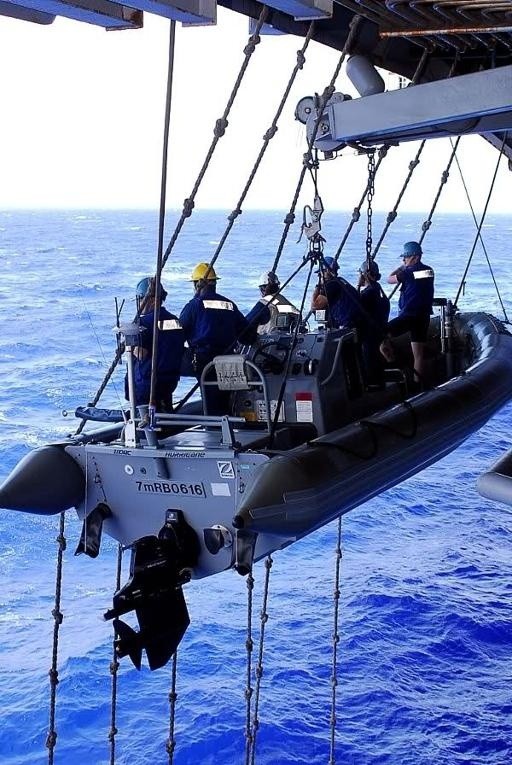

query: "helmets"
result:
(257, 270), (279, 287)
(186, 262), (222, 282)
(356, 261), (381, 280)
(399, 242), (423, 257)
(314, 257), (339, 274)
(131, 277), (167, 303)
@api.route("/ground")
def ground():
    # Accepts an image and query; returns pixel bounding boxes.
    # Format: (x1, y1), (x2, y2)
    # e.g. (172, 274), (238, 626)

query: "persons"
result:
(357, 260), (390, 353)
(310, 257), (359, 330)
(124, 278), (185, 413)
(244, 271), (294, 327)
(386, 241), (434, 375)
(179, 263), (255, 416)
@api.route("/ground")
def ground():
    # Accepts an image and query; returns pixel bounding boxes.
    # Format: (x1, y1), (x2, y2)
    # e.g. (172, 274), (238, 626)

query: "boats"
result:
(0, 311), (512, 671)
